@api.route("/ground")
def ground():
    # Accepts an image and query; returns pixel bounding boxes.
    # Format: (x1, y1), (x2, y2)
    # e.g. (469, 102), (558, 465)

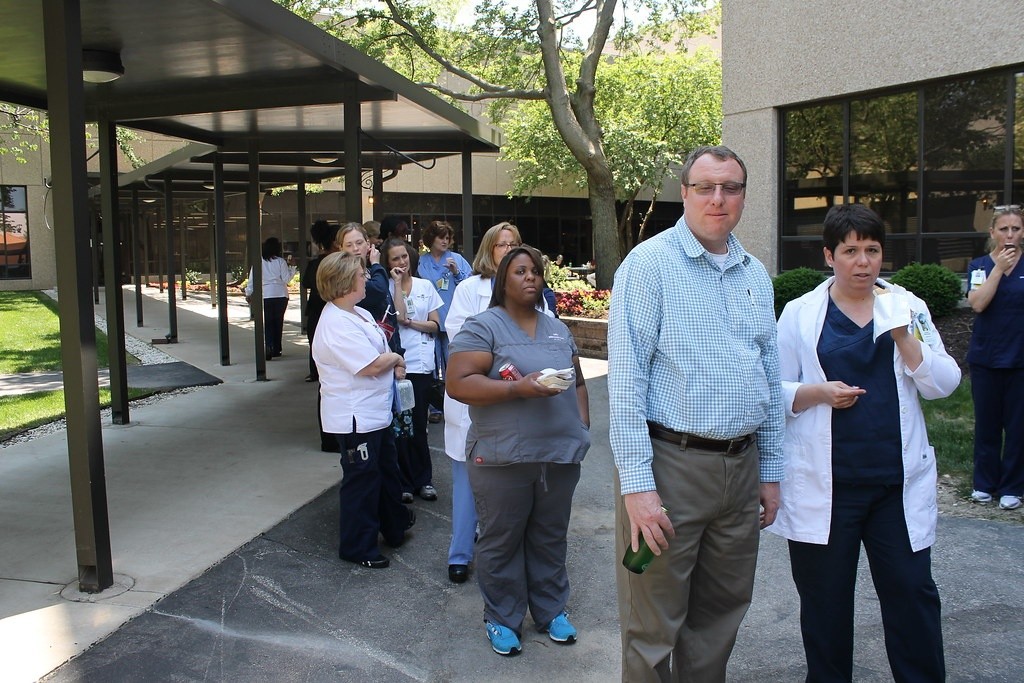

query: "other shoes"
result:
(356, 554), (389, 568)
(449, 565), (467, 582)
(388, 510), (415, 548)
(429, 413), (442, 423)
(304, 375), (315, 382)
(273, 352), (281, 357)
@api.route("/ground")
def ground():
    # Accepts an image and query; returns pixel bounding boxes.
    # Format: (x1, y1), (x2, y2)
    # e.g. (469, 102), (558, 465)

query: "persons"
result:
(418, 239), (430, 255)
(246, 237), (297, 361)
(333, 222), (406, 358)
(606, 145), (787, 683)
(380, 238), (445, 501)
(311, 252), (416, 568)
(303, 220), (340, 382)
(553, 255), (566, 268)
(445, 247), (591, 655)
(759, 203), (961, 683)
(964, 205), (1024, 510)
(364, 214), (419, 277)
(417, 221), (473, 423)
(443, 222), (555, 583)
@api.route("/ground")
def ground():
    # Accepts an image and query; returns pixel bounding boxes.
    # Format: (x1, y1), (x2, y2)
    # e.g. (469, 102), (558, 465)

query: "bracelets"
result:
(404, 318), (411, 327)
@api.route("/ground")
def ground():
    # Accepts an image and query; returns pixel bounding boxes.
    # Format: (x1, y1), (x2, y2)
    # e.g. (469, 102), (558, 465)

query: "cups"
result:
(375, 320), (395, 343)
(288, 255), (292, 262)
(569, 262), (572, 267)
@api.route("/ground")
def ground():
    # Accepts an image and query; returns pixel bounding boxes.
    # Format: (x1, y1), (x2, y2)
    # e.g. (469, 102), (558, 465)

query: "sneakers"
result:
(401, 493), (414, 502)
(544, 612), (577, 642)
(486, 620), (522, 655)
(999, 495), (1022, 509)
(419, 485), (438, 499)
(972, 491), (992, 502)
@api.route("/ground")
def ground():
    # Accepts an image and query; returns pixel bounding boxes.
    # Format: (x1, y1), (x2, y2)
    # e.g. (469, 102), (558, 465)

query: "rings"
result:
(403, 374), (405, 377)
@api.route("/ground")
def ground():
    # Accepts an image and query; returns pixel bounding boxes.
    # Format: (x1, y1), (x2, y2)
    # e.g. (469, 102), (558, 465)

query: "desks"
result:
(570, 267), (595, 281)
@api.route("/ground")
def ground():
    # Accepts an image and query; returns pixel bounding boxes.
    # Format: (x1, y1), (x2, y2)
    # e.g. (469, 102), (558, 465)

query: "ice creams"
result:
(1004, 242), (1015, 251)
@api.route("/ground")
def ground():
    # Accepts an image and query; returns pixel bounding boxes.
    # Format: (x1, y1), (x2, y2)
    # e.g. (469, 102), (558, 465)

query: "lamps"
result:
(83, 49), (125, 83)
(263, 188), (273, 196)
(311, 153), (338, 163)
(202, 182), (214, 189)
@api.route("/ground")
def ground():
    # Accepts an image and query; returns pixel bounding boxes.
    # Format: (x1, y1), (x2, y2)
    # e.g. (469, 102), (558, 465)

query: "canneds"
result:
(499, 363), (523, 381)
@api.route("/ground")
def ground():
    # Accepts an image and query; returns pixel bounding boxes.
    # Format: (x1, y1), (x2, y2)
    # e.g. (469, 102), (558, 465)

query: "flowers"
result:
(553, 288), (611, 319)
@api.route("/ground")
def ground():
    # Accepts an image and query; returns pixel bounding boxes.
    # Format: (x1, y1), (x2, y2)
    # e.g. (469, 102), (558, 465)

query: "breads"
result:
(872, 288), (892, 296)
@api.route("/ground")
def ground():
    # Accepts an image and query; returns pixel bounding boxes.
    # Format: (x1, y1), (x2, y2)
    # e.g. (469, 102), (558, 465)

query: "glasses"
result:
(992, 204), (1022, 213)
(494, 243), (519, 251)
(685, 180), (744, 196)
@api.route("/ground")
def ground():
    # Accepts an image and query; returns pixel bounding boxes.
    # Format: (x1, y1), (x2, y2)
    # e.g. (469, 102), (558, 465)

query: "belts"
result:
(646, 419), (756, 456)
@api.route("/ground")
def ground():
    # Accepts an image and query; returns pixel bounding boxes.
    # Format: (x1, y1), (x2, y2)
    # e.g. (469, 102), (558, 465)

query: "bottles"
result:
(621, 507), (667, 574)
